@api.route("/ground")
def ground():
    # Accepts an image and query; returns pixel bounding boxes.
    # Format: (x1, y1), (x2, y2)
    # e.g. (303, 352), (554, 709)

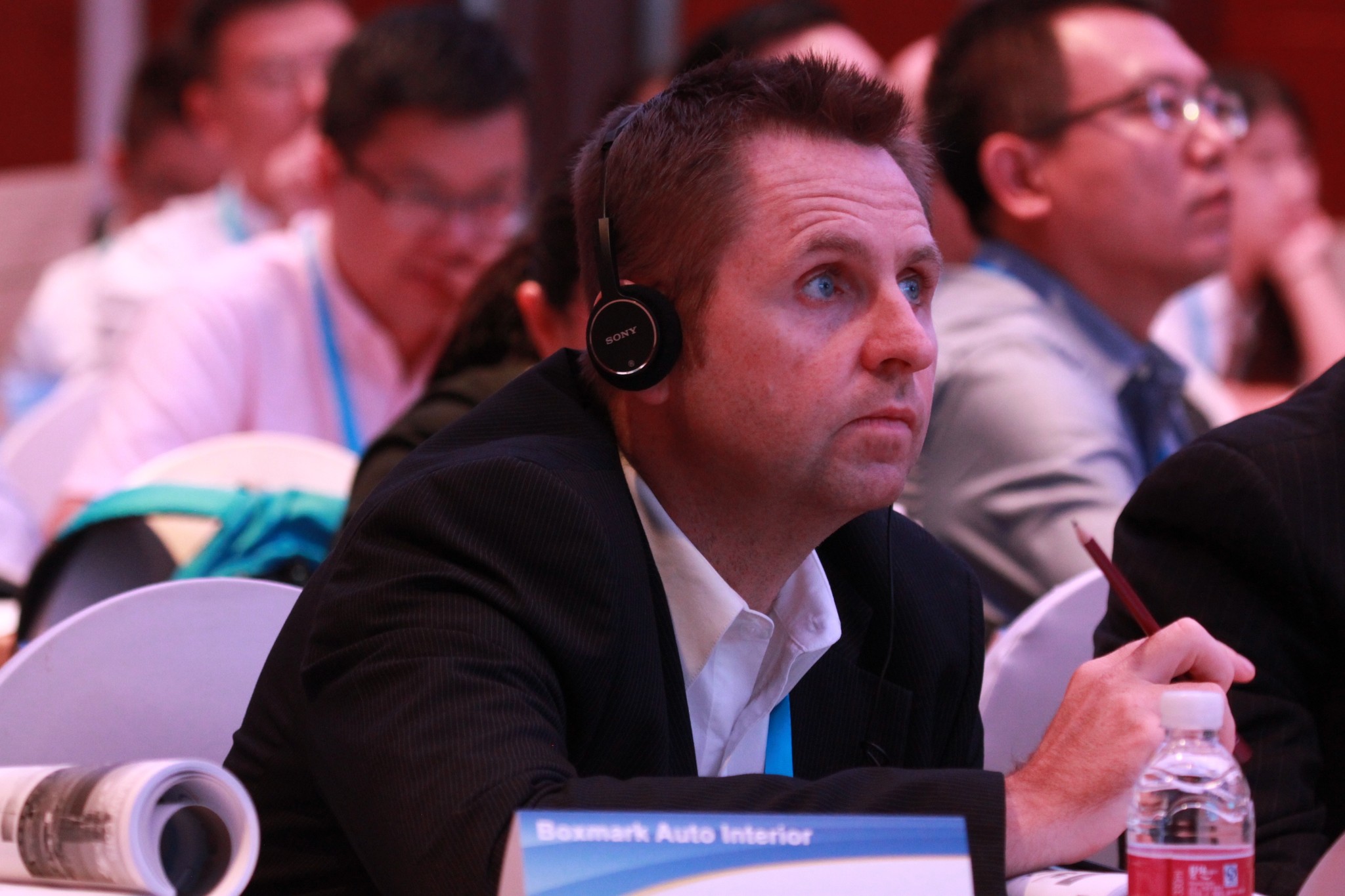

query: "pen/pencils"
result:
(1070, 520), (1254, 764)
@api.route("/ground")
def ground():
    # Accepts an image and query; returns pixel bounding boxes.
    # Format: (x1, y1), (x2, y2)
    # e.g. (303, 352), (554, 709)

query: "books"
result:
(0, 758), (260, 896)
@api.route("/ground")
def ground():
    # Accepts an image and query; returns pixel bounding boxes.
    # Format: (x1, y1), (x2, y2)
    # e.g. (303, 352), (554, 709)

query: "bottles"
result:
(1125, 690), (1254, 896)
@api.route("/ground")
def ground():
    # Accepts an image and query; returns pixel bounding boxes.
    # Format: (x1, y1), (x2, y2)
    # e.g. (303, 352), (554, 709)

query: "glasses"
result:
(1024, 76), (1254, 145)
(343, 163), (510, 230)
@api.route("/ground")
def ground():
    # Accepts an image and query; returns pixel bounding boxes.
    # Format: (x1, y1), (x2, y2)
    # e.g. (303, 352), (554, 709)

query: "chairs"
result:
(1, 575), (301, 768)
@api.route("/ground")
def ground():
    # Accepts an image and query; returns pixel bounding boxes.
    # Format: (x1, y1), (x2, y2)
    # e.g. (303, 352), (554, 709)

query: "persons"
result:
(0, 0), (1345, 896)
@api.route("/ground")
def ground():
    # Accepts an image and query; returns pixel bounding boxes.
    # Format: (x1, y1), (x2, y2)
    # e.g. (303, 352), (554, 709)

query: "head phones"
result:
(587, 112), (681, 391)
